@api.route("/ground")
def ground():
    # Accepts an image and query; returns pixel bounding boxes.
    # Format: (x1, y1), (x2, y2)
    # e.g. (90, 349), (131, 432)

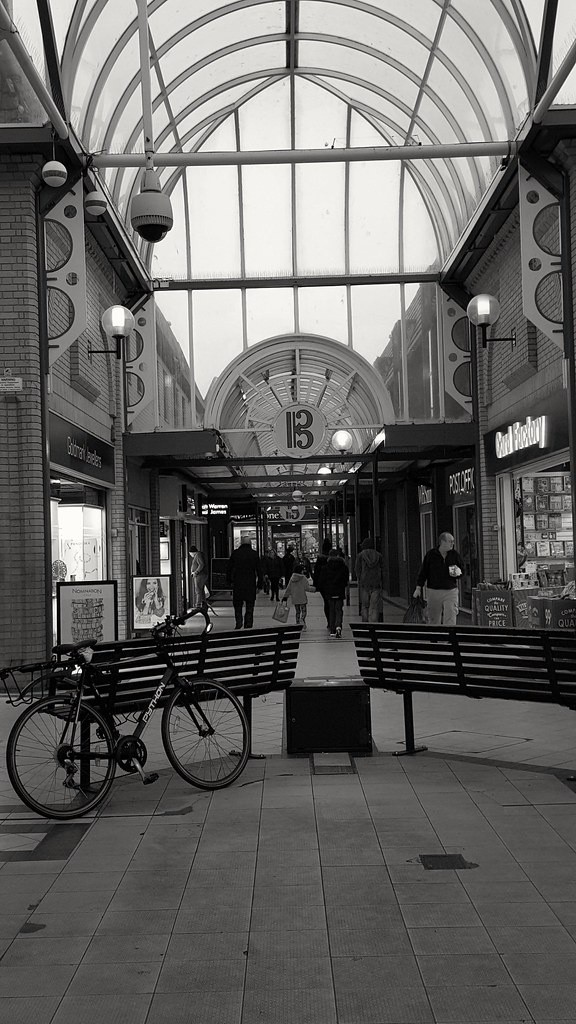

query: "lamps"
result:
(41, 133), (67, 187)
(88, 305), (135, 360)
(466, 294), (516, 348)
(84, 176), (107, 215)
(317, 453), (353, 486)
(332, 429), (371, 465)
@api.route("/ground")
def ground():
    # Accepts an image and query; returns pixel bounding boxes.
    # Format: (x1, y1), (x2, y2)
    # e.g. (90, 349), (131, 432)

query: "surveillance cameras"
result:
(130, 170), (173, 244)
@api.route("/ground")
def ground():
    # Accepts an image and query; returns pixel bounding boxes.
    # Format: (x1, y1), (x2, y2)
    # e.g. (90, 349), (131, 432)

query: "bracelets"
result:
(416, 586), (422, 590)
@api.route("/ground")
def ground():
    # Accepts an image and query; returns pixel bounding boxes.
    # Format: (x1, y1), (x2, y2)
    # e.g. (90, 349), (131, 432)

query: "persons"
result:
(188, 546), (213, 635)
(354, 538), (384, 622)
(313, 538), (332, 592)
(281, 564), (316, 632)
(264, 548), (283, 601)
(226, 536), (263, 630)
(413, 532), (465, 645)
(316, 549), (349, 638)
(135, 579), (164, 615)
(336, 548), (350, 606)
(281, 545), (297, 587)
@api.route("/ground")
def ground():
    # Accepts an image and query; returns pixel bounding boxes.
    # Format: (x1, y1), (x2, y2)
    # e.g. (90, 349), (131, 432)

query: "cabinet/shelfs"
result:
(517, 492), (574, 560)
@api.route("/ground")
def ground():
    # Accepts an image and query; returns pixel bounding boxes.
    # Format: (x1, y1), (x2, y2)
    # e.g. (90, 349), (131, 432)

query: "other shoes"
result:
(234, 623), (243, 630)
(300, 618), (307, 629)
(336, 627), (342, 639)
(329, 632), (336, 637)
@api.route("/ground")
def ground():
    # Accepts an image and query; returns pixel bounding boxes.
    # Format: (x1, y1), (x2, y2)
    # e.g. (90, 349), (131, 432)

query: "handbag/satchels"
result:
(404, 596), (427, 625)
(272, 599), (290, 623)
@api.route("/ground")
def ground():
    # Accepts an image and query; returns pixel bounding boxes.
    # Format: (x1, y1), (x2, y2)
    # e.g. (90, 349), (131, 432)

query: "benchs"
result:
(37, 623), (304, 795)
(350, 620), (576, 781)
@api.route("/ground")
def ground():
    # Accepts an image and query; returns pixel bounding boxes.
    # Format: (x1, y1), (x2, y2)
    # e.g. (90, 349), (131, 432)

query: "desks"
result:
(286, 680), (373, 755)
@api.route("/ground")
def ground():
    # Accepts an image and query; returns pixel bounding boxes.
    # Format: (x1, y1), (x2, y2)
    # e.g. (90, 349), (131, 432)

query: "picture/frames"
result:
(159, 542), (170, 560)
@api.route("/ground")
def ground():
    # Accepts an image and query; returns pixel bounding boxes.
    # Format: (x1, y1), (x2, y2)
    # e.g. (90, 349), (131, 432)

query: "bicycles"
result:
(0, 593), (252, 821)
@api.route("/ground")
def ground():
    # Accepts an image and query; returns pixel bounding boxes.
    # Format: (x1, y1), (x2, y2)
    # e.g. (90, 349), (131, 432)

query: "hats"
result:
(361, 538), (373, 549)
(189, 545), (198, 552)
